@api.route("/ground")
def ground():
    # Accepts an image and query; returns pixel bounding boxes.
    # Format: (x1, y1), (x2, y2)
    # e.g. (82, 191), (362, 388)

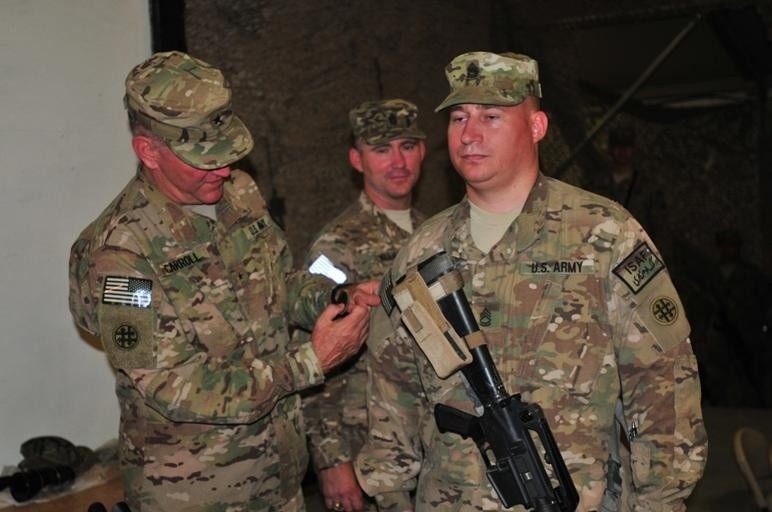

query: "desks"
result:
(0, 454), (128, 511)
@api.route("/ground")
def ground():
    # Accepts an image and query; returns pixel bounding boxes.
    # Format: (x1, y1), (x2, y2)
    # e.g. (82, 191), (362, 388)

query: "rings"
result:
(333, 501), (341, 510)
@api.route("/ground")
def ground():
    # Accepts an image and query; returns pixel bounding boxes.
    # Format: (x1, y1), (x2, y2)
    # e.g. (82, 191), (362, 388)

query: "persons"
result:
(352, 52), (709, 510)
(67, 51), (381, 510)
(296, 97), (434, 510)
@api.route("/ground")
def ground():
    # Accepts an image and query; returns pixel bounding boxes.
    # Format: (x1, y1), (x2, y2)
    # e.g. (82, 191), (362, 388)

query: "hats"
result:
(432, 50), (544, 116)
(122, 49), (255, 171)
(346, 95), (427, 147)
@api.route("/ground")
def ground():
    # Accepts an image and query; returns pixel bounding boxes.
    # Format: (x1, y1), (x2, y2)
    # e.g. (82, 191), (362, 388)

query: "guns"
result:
(391, 251), (580, 511)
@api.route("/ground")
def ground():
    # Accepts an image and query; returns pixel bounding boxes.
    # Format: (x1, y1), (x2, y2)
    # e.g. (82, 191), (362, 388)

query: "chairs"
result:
(733, 425), (772, 511)
